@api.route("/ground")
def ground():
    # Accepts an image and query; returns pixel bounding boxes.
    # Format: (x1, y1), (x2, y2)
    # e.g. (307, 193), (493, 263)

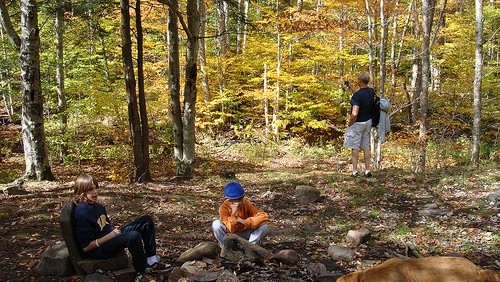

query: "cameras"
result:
(343, 81), (349, 87)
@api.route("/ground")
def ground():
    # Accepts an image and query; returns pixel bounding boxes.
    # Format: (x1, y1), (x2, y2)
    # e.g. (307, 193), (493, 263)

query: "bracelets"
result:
(96, 239), (100, 247)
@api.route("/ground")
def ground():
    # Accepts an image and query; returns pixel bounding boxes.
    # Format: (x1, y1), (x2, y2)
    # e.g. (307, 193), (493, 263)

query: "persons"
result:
(73, 174), (173, 282)
(212, 182), (270, 257)
(343, 74), (375, 178)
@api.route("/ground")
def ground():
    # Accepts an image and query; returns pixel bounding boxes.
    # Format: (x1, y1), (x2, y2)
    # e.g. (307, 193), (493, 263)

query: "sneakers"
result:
(148, 262), (173, 273)
(135, 272), (156, 282)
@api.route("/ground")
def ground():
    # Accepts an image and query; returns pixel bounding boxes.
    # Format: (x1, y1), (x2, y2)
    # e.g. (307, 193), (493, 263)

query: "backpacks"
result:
(371, 87), (380, 127)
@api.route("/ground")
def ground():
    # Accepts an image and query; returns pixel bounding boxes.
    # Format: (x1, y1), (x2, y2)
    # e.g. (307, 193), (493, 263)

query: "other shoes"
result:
(350, 171), (360, 178)
(364, 172), (372, 178)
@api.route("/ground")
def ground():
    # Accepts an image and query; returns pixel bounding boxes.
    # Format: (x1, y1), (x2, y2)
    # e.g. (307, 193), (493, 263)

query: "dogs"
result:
(336, 256), (500, 282)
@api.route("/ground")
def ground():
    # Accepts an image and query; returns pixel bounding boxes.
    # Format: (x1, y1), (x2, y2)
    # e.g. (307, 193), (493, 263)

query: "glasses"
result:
(232, 198), (243, 202)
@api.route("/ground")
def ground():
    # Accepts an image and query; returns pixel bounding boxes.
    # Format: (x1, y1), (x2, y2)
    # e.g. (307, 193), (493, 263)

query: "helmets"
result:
(224, 182), (246, 200)
